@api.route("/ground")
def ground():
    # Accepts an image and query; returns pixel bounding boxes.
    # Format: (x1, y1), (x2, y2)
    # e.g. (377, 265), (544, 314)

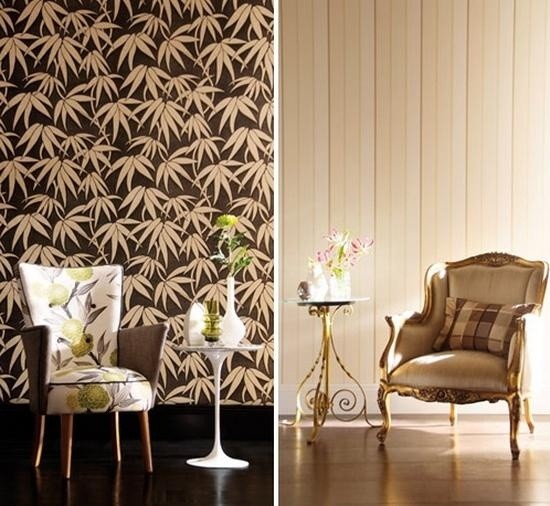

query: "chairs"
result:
(374, 252), (550, 463)
(10, 259), (171, 481)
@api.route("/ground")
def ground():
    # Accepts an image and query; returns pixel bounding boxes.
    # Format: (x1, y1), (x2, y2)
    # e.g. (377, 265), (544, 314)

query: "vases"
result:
(216, 276), (245, 344)
(309, 266), (329, 298)
(330, 271), (351, 297)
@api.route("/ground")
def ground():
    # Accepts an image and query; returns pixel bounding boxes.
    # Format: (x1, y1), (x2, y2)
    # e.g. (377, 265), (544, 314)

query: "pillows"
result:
(434, 290), (540, 358)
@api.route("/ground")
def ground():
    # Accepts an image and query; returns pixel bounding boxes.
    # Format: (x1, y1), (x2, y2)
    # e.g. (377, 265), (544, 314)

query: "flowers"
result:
(307, 227), (374, 290)
(205, 213), (255, 278)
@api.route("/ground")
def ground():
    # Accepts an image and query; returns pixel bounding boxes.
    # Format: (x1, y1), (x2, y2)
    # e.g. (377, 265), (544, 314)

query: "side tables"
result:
(175, 346), (264, 469)
(285, 298), (383, 445)
